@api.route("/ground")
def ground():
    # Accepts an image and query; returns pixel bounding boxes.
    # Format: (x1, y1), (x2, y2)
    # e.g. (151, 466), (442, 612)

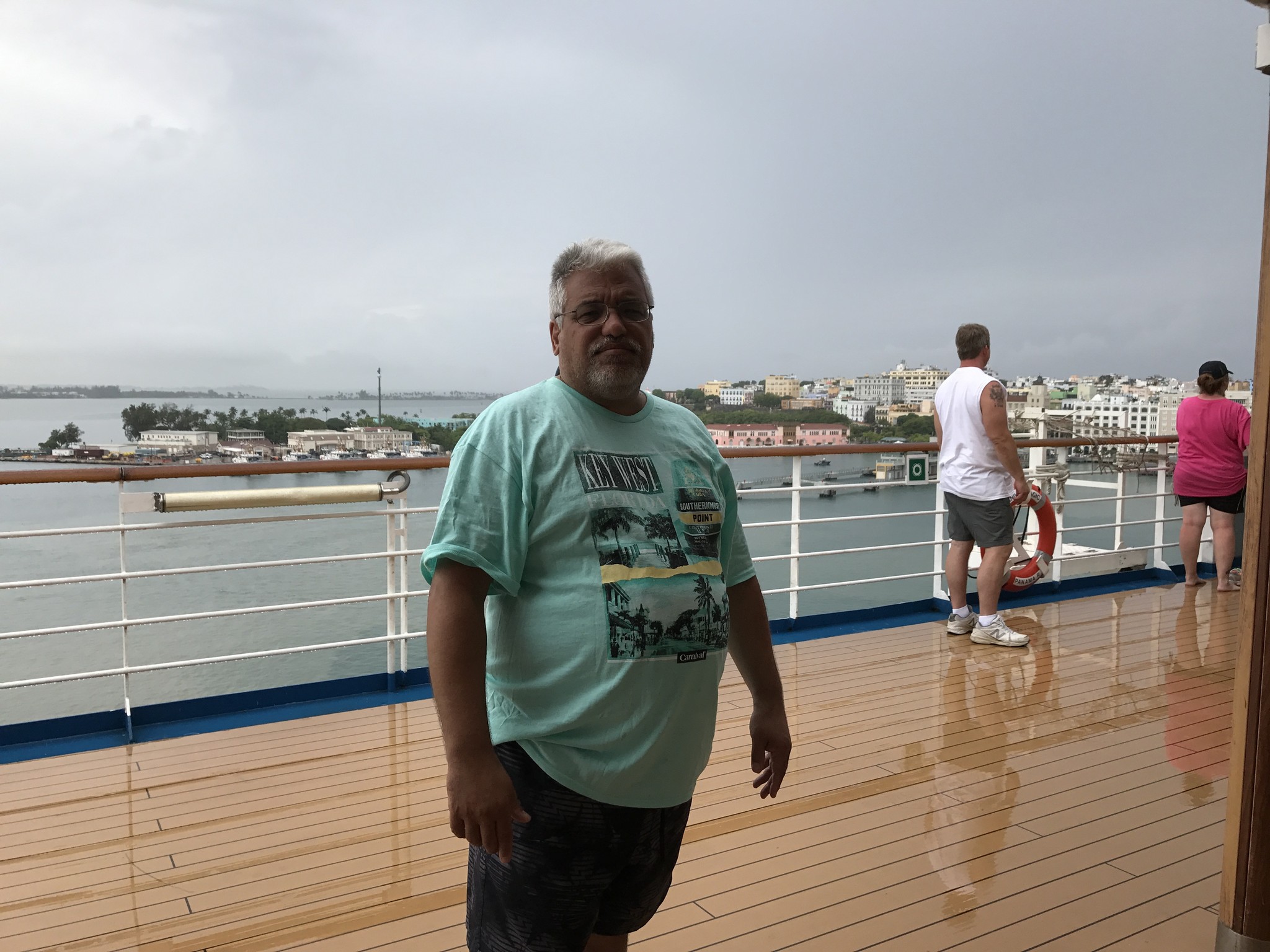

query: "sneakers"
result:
(946, 605), (979, 634)
(1228, 567), (1241, 585)
(970, 614), (1031, 647)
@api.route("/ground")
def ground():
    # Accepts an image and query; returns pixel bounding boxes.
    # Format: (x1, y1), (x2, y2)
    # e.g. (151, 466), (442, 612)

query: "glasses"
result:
(554, 299), (656, 327)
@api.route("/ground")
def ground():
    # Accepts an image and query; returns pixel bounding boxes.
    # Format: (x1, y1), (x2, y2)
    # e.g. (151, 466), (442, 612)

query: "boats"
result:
(814, 461), (831, 466)
(231, 447), (432, 463)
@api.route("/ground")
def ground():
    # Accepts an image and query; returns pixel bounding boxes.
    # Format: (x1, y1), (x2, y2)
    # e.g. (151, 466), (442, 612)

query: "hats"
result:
(1198, 360), (1236, 379)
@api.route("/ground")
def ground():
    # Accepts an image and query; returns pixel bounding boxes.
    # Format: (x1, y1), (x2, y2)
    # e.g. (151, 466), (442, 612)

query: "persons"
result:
(1172, 361), (1252, 592)
(930, 324), (1030, 647)
(420, 238), (792, 952)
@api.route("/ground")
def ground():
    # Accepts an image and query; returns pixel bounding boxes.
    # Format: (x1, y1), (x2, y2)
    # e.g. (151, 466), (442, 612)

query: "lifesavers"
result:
(980, 484), (1057, 591)
